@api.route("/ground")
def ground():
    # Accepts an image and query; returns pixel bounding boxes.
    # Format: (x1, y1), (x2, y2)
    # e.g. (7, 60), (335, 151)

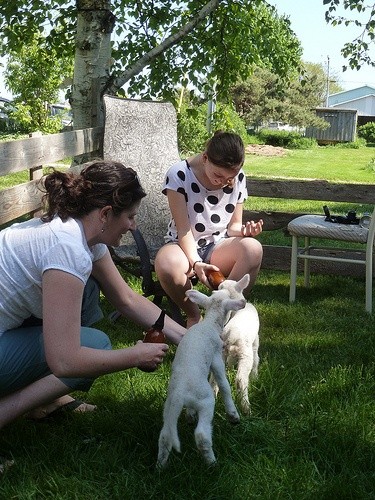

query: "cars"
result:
(50, 103), (73, 127)
(263, 121), (287, 132)
(0, 96), (31, 129)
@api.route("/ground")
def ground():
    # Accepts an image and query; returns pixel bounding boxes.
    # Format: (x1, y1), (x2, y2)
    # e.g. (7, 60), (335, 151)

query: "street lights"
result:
(324, 55), (331, 106)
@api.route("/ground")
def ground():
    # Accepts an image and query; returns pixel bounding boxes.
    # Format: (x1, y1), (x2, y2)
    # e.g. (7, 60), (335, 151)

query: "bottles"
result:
(206, 270), (226, 290)
(137, 309), (167, 373)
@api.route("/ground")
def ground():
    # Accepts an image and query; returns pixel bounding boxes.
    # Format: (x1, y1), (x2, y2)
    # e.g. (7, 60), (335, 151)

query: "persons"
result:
(0, 160), (189, 428)
(155, 130), (264, 328)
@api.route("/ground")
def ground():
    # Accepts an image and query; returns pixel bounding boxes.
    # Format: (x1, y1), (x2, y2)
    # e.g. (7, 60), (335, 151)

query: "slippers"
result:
(36, 401), (92, 422)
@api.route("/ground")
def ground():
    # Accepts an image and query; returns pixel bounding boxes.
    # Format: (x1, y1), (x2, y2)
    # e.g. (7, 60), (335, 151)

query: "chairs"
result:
(287, 204), (375, 312)
(102, 94), (199, 327)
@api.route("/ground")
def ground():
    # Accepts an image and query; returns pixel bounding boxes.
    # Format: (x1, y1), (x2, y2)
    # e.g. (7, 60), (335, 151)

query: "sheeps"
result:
(155, 288), (246, 472)
(212, 274), (260, 414)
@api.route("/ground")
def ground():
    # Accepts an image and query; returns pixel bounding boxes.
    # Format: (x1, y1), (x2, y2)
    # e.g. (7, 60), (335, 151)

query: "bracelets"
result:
(193, 261), (204, 275)
